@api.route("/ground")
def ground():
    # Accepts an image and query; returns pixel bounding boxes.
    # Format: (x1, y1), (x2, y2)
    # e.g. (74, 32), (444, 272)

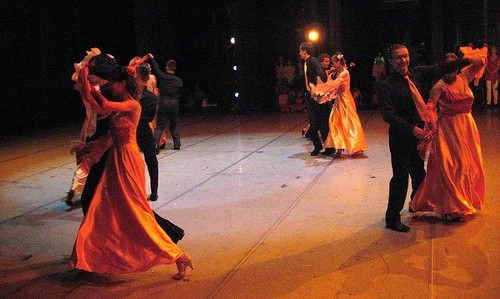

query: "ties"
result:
(404, 74), (432, 123)
(304, 61), (311, 91)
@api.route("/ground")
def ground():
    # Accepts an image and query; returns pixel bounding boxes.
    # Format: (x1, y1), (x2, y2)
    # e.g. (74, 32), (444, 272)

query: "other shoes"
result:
(150, 193), (158, 201)
(387, 222), (410, 232)
(310, 148), (336, 156)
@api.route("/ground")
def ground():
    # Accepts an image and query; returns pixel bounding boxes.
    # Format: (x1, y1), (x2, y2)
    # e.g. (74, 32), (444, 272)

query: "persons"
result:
(69, 65), (195, 280)
(128, 65), (160, 201)
(305, 54), (331, 142)
(485, 45), (500, 111)
(66, 47), (111, 207)
(299, 42), (337, 157)
(301, 53), (348, 138)
(177, 41), (487, 120)
(125, 54), (167, 150)
(409, 47), (487, 225)
(70, 53), (185, 244)
(309, 51), (369, 157)
(378, 43), (485, 232)
(150, 52), (184, 153)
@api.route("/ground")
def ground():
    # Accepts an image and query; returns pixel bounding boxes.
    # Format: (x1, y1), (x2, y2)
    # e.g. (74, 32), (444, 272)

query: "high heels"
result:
(171, 253), (194, 280)
(335, 149), (363, 157)
